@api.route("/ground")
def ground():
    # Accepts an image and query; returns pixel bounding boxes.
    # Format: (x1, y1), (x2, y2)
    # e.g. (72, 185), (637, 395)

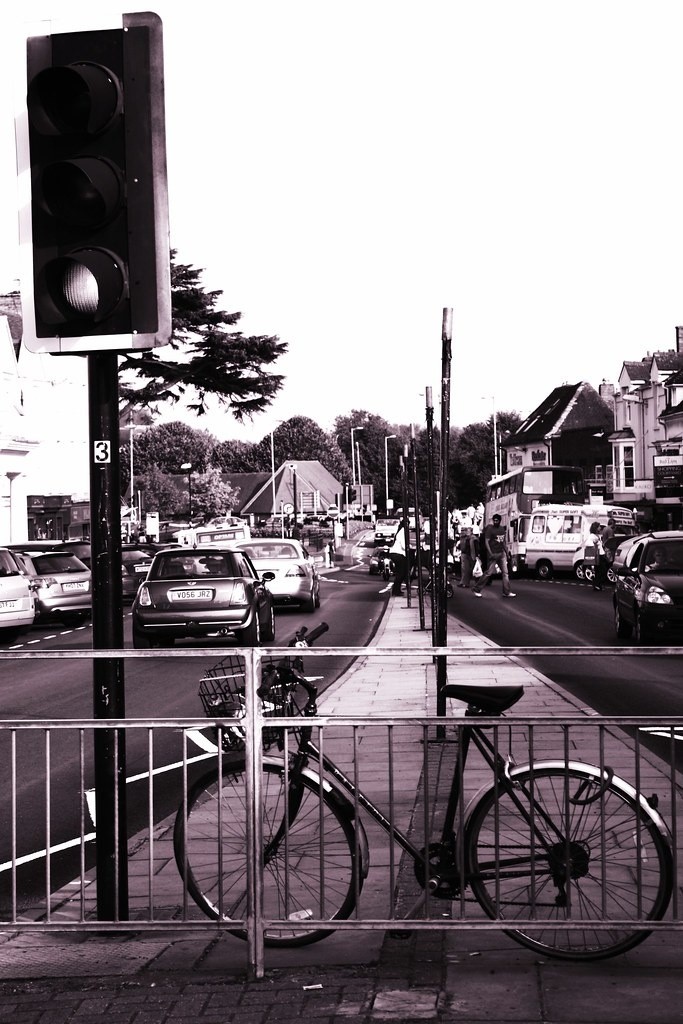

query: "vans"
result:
(524, 504), (642, 579)
(399, 507), (424, 530)
(506, 512), (532, 574)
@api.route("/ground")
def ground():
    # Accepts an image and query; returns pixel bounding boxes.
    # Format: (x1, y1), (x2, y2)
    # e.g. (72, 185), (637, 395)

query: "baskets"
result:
(198, 655), (297, 750)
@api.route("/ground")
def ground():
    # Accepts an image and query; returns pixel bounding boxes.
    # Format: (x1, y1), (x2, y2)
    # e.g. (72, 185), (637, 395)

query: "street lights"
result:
(182, 463), (192, 515)
(350, 426), (365, 486)
(385, 435), (396, 517)
(270, 418), (284, 514)
(482, 396), (510, 477)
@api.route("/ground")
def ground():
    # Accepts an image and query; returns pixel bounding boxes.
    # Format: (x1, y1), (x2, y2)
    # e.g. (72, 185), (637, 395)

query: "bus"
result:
(484, 465), (586, 527)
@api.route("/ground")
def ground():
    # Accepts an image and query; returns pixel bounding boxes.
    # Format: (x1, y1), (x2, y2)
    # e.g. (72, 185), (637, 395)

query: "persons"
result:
(420, 501), (485, 545)
(457, 513), (516, 597)
(133, 527), (157, 543)
(585, 518), (616, 590)
(389, 519), (411, 596)
(645, 548), (672, 572)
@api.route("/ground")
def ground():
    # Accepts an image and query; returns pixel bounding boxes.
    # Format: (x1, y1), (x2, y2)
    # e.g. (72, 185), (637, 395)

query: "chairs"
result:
(162, 562), (185, 576)
(244, 548), (255, 557)
(281, 547), (292, 556)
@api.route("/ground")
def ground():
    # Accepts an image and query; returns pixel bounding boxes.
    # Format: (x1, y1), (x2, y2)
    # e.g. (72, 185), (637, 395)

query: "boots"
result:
(391, 584), (404, 596)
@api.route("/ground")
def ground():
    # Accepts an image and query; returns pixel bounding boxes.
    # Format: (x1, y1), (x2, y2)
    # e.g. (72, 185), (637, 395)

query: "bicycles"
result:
(173, 619), (676, 961)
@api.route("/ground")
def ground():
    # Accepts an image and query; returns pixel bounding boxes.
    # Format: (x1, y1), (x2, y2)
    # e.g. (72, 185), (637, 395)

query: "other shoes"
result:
(456, 584), (469, 588)
(503, 592), (516, 597)
(470, 589), (482, 597)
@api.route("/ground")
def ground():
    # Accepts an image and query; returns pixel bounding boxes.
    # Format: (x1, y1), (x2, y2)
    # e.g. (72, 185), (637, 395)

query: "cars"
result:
(138, 515), (333, 570)
(238, 539), (321, 613)
(131, 546), (277, 650)
(0, 543), (190, 638)
(571, 533), (642, 583)
(612, 531), (683, 646)
(368, 519), (405, 579)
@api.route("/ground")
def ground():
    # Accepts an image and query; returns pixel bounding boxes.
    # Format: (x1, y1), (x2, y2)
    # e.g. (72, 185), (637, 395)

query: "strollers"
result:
(416, 547), (454, 600)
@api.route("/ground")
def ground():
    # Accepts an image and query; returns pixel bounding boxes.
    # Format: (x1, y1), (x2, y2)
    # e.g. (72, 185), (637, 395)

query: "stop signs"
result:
(328, 506), (339, 517)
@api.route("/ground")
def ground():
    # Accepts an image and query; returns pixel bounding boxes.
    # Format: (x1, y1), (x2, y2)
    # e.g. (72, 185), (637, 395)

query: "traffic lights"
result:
(15, 13), (174, 354)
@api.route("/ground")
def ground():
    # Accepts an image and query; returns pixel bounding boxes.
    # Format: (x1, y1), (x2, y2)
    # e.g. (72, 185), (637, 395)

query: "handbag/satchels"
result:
(473, 556), (483, 578)
